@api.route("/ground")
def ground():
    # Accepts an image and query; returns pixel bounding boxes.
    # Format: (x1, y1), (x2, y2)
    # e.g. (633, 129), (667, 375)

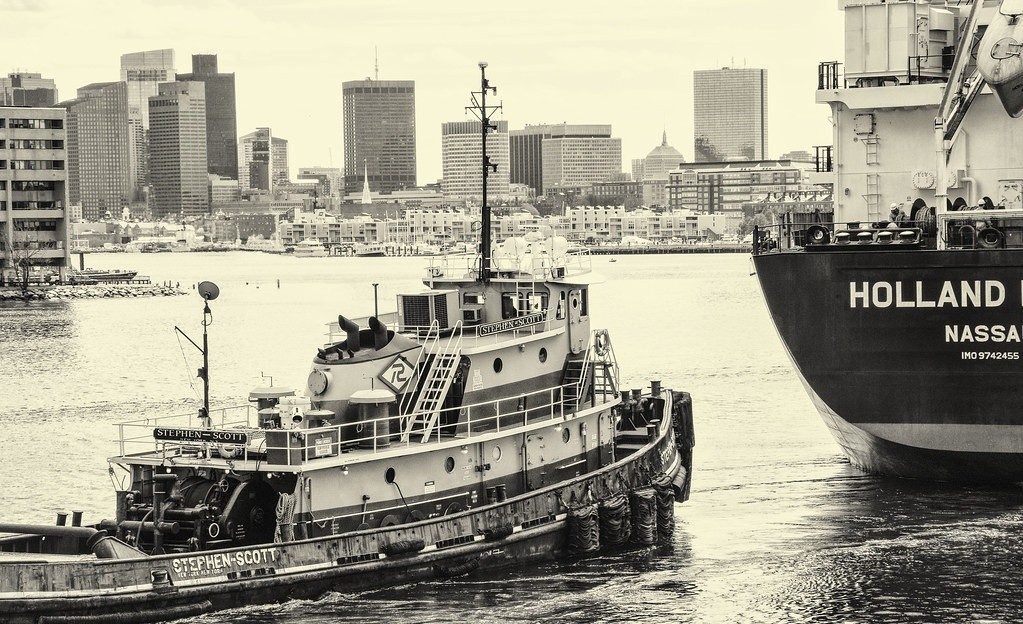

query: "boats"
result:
(747, 0), (1022, 468)
(0, 58), (698, 624)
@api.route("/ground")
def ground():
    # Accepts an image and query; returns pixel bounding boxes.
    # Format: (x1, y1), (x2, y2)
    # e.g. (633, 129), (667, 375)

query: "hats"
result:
(978, 199), (986, 204)
(890, 203), (897, 210)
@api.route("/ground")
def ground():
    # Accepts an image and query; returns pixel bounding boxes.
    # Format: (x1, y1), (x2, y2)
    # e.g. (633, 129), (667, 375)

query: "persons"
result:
(975, 199), (991, 230)
(888, 203), (907, 227)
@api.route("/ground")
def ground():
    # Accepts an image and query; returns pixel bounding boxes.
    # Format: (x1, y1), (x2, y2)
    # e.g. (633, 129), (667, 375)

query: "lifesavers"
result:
(216, 427), (241, 459)
(595, 330), (611, 357)
(977, 226), (1002, 249)
(958, 224), (976, 245)
(806, 224), (830, 244)
